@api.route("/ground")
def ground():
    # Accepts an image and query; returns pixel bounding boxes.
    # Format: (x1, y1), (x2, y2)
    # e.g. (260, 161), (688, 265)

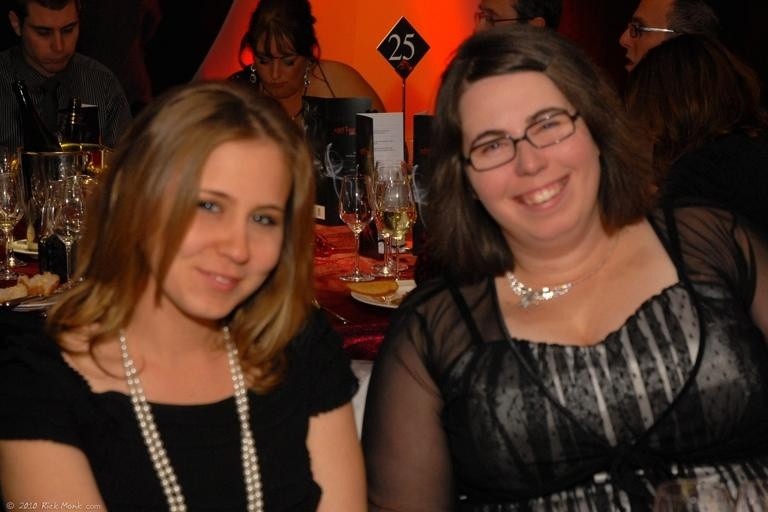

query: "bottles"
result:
(12, 79), (79, 149)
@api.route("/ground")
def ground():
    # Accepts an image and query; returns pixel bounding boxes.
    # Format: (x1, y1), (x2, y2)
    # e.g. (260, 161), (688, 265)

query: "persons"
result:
(475, 0), (564, 29)
(0, 0), (132, 172)
(628, 37), (768, 236)
(618, 0), (706, 73)
(1, 80), (370, 512)
(215, 0), (388, 155)
(338, 30), (768, 512)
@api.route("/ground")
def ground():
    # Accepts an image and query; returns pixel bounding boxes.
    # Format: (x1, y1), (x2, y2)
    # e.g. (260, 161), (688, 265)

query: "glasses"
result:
(628, 25), (678, 37)
(460, 108), (585, 174)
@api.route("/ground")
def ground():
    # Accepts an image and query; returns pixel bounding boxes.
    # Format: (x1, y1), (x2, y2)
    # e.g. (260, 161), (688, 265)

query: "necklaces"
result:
(287, 80), (313, 120)
(503, 228), (624, 309)
(107, 313), (265, 512)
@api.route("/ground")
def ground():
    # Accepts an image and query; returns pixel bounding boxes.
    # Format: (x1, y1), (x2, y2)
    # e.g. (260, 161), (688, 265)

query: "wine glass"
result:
(0, 169), (84, 291)
(339, 162), (418, 282)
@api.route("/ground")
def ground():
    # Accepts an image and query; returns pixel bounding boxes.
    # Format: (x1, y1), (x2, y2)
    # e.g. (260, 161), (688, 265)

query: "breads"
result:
(0, 272), (59, 303)
(346, 278), (398, 296)
